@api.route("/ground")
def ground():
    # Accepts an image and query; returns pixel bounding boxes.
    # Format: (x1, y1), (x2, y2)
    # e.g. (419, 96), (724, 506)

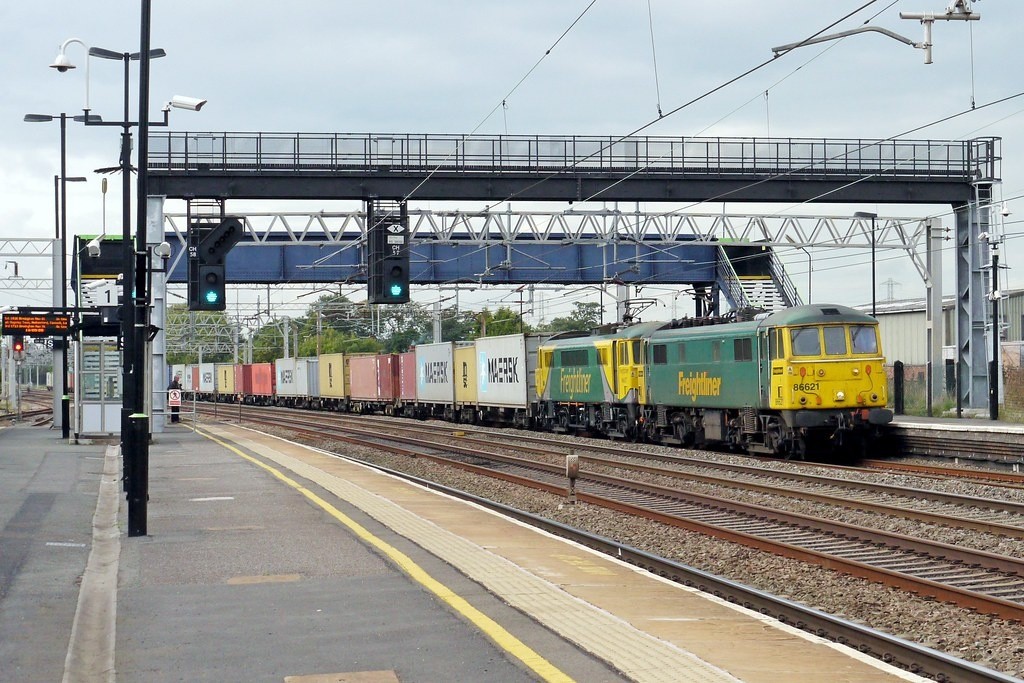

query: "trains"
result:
(170, 304), (893, 459)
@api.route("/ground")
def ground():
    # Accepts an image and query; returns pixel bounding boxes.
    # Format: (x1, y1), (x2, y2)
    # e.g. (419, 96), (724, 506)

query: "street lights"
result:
(54, 175), (87, 239)
(48, 38), (207, 494)
(783, 234), (811, 304)
(24, 113), (104, 441)
(854, 211), (878, 319)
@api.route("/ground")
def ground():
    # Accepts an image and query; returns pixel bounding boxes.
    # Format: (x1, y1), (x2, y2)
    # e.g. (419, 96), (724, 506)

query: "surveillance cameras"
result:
(977, 232), (985, 240)
(171, 95), (207, 111)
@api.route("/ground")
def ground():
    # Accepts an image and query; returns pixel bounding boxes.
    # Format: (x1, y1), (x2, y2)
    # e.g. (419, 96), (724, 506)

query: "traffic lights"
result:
(197, 263), (227, 310)
(12, 335), (24, 351)
(197, 217), (244, 264)
(382, 255), (411, 303)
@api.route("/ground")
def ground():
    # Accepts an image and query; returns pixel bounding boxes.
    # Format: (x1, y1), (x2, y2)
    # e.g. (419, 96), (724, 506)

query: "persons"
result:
(25, 380), (32, 393)
(167, 375), (182, 424)
